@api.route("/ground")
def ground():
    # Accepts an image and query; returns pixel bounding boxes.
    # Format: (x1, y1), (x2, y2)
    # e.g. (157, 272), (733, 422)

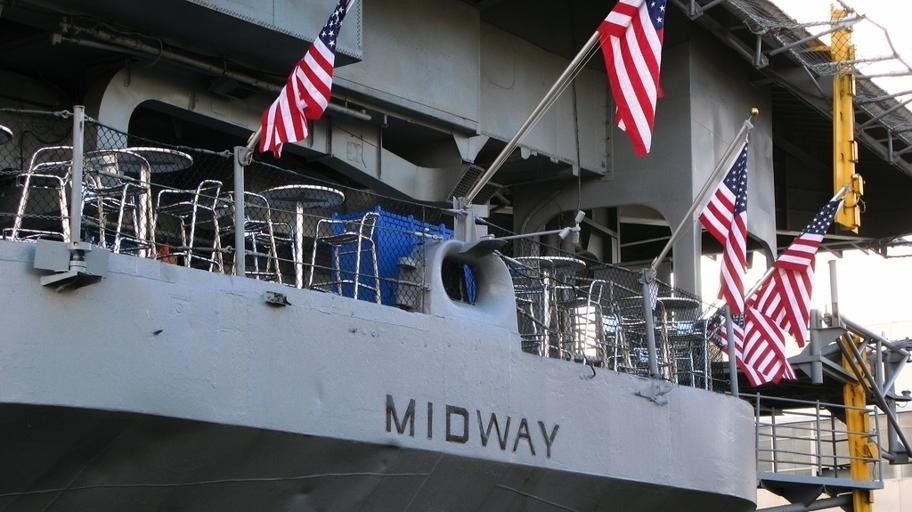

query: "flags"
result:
(259, 0), (354, 161)
(596, 0), (668, 158)
(692, 198), (844, 389)
(697, 133), (748, 335)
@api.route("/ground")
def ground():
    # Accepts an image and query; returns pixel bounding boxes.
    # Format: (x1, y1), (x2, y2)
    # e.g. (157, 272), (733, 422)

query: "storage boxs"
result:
(329, 206), (476, 314)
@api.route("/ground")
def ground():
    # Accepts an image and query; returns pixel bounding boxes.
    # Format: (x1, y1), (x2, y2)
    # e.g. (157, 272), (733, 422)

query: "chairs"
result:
(224, 180), (381, 303)
(7, 129), (224, 278)
(510, 249), (707, 388)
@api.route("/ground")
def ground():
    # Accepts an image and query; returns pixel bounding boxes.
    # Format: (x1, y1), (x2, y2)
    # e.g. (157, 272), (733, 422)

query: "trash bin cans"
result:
(565, 306), (597, 365)
